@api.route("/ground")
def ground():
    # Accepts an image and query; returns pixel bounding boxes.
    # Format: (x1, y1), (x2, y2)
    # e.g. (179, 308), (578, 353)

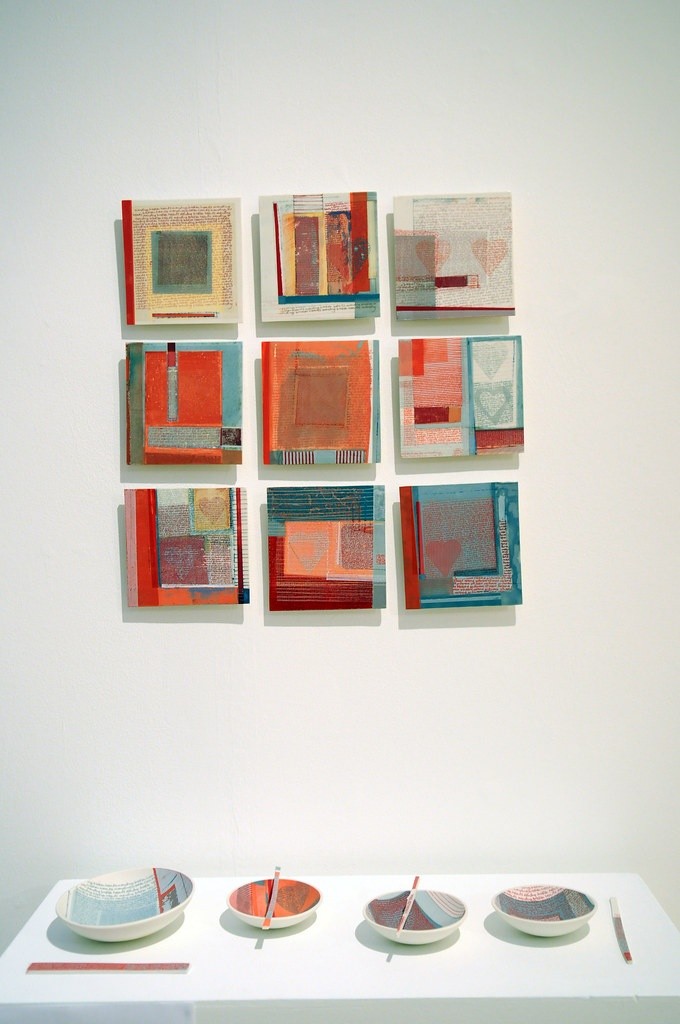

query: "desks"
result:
(0, 874), (680, 1024)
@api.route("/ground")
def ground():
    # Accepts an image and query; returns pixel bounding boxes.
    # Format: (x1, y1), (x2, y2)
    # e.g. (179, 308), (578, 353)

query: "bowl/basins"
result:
(362, 890), (467, 946)
(492, 884), (598, 936)
(56, 867), (194, 942)
(227, 880), (322, 928)
